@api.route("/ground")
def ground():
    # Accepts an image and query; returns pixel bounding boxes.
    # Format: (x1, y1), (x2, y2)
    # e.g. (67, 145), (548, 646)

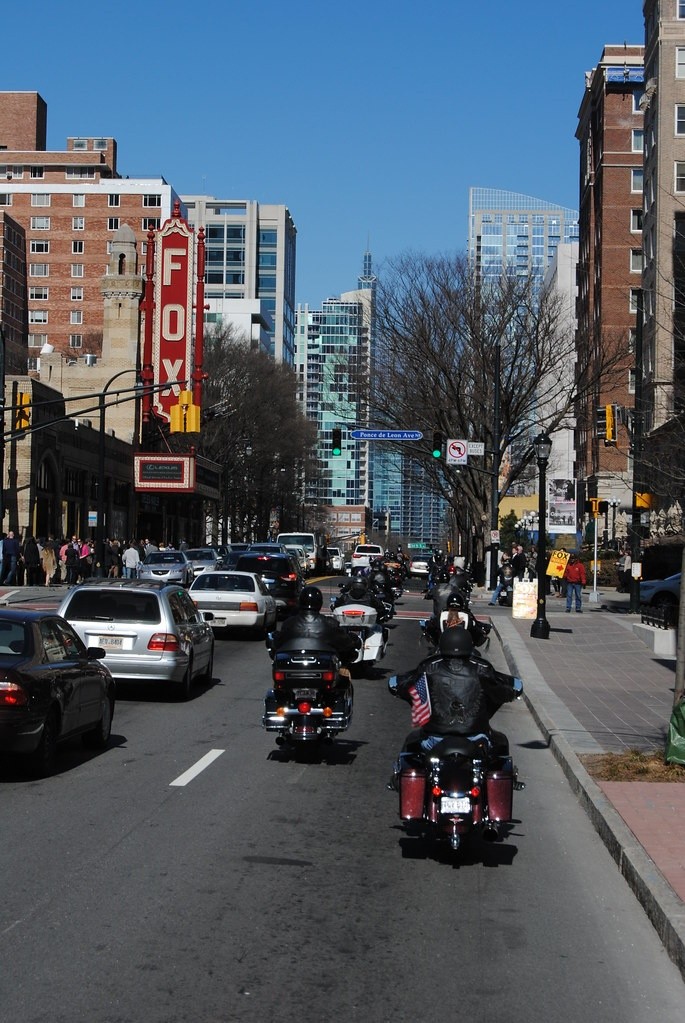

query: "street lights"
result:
(94, 364), (155, 578)
(530, 429), (554, 639)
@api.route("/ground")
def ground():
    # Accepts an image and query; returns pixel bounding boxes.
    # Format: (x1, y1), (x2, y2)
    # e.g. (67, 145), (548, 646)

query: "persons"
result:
(317, 542), (333, 574)
(547, 473), (573, 528)
(420, 549), (491, 658)
(0, 527), (200, 590)
(267, 586), (362, 708)
(335, 543), (410, 651)
(612, 548), (645, 593)
(488, 537), (588, 613)
(388, 626), (524, 793)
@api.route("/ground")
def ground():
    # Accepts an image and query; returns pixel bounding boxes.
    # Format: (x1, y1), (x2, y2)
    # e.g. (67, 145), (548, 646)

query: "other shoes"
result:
(576, 610), (583, 613)
(565, 610), (571, 613)
(488, 603), (495, 606)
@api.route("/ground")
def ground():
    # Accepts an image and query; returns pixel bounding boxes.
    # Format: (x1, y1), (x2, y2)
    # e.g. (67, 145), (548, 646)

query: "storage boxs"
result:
(364, 629), (383, 661)
(485, 768), (513, 823)
(273, 648), (339, 693)
(333, 604), (379, 628)
(398, 767), (425, 822)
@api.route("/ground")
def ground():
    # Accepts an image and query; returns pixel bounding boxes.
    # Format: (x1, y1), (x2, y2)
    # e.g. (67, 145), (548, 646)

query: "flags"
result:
(409, 674), (432, 729)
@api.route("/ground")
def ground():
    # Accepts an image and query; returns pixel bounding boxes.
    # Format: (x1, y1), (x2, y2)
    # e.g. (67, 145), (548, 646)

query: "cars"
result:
(137, 548), (224, 586)
(187, 570), (277, 636)
(408, 554), (432, 577)
(0, 608), (117, 772)
(638, 572), (680, 608)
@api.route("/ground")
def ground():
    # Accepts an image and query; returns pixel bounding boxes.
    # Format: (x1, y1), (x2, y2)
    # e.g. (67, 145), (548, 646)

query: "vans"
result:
(278, 531), (324, 573)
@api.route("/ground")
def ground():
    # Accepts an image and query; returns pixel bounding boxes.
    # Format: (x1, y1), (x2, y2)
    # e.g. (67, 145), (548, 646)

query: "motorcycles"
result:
(328, 551), (493, 678)
(388, 673), (523, 864)
(262, 631), (364, 767)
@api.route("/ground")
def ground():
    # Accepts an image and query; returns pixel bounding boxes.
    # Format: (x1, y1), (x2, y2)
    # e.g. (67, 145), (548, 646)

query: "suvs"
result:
(57, 578), (215, 702)
(222, 542), (307, 622)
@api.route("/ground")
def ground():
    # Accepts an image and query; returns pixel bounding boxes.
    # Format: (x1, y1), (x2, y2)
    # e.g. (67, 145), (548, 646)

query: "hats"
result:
(570, 553), (578, 559)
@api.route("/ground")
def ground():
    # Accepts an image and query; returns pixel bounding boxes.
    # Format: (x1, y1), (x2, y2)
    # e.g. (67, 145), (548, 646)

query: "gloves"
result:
(582, 584), (586, 589)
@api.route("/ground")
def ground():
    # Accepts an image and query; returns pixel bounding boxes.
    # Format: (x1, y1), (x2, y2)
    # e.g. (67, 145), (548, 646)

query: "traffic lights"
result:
(0, 397), (6, 423)
(446, 544), (449, 552)
(585, 497), (609, 516)
(332, 428), (341, 455)
(432, 432), (443, 458)
(595, 408), (607, 440)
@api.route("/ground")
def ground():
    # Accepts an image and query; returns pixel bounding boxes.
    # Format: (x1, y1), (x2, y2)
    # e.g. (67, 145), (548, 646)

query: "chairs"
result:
(8, 640), (25, 653)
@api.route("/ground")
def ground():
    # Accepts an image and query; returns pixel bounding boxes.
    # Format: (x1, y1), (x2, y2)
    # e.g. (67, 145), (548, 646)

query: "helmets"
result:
(298, 586), (323, 609)
(435, 555), (441, 561)
(352, 576), (368, 590)
(373, 560), (387, 572)
(447, 594), (464, 609)
(353, 567), (366, 577)
(449, 567), (457, 574)
(440, 627), (472, 656)
(440, 572), (450, 584)
